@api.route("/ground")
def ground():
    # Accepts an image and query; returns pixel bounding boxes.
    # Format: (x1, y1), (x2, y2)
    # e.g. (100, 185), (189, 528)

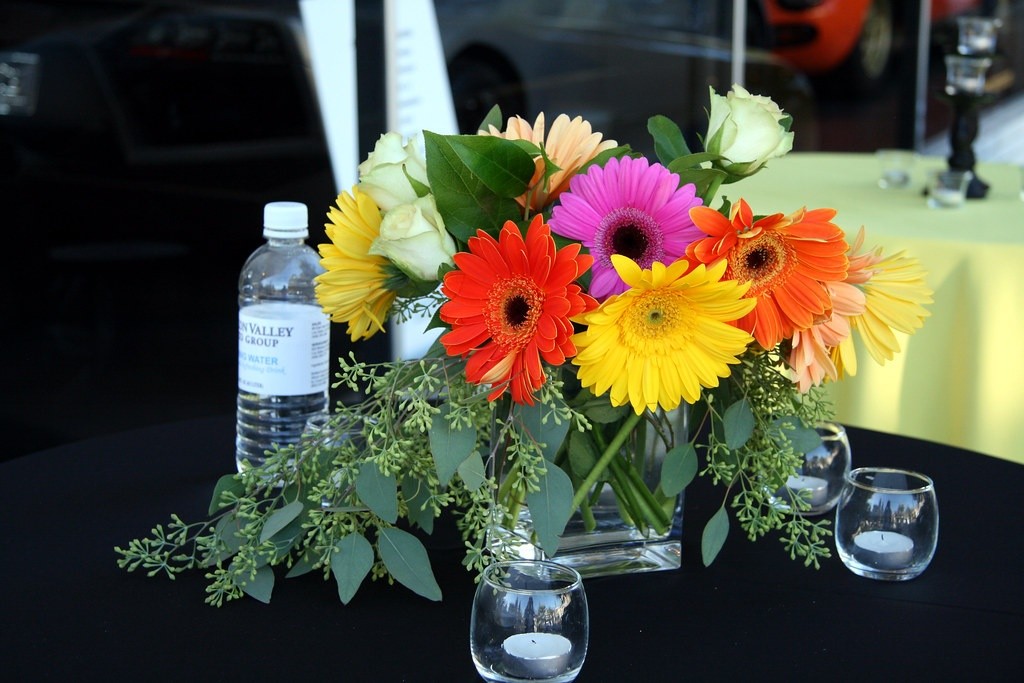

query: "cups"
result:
(470, 561), (589, 683)
(835, 467), (940, 580)
(306, 415), (378, 507)
(758, 420), (852, 515)
(928, 171), (973, 208)
(876, 148), (917, 189)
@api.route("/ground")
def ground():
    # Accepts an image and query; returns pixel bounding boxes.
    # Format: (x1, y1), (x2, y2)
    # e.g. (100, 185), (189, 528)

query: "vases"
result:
(483, 395), (687, 576)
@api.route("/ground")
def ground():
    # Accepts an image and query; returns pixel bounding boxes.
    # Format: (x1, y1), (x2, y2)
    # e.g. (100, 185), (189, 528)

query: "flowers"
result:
(96, 81), (942, 609)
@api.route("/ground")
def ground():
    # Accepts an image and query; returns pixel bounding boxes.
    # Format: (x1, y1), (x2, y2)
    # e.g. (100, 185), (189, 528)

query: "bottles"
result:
(236, 201), (331, 487)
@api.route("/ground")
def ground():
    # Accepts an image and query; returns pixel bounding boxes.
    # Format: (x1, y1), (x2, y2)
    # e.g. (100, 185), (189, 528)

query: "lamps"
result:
(926, 7), (1006, 197)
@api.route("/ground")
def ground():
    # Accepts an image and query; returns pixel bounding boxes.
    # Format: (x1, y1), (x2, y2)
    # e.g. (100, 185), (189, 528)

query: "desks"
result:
(0, 388), (1024, 680)
(707, 150), (1024, 465)
